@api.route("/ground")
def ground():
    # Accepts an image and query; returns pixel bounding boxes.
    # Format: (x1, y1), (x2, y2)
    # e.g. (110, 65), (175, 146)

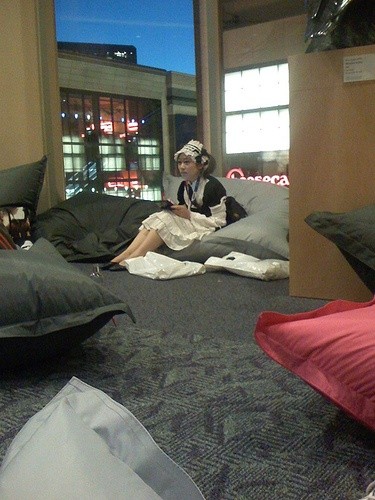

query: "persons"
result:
(97, 139), (247, 272)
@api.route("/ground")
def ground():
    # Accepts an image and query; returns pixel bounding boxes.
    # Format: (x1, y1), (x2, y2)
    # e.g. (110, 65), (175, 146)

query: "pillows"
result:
(0, 237), (136, 353)
(256, 294), (375, 431)
(0, 376), (206, 500)
(0, 155), (48, 250)
(304, 205), (375, 293)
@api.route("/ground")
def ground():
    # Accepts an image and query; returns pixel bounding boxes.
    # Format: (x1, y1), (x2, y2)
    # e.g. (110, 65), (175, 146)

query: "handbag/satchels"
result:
(118, 251), (207, 280)
(204, 251), (290, 282)
(215, 197), (248, 231)
